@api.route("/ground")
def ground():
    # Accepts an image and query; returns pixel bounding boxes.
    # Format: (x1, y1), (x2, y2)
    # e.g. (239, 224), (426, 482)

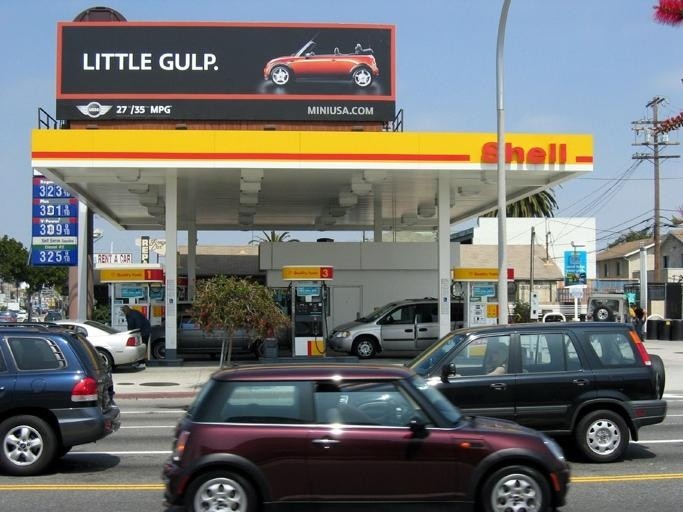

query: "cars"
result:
(51, 320), (148, 368)
(163, 364), (571, 512)
(0, 280), (68, 325)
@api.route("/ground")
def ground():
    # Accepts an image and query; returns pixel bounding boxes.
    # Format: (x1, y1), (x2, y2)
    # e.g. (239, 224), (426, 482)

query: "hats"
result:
(119, 305), (129, 310)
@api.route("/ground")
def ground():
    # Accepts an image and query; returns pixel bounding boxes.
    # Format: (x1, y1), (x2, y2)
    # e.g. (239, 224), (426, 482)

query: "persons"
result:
(119, 304), (152, 369)
(315, 384), (376, 425)
(482, 341), (510, 375)
(630, 304), (645, 342)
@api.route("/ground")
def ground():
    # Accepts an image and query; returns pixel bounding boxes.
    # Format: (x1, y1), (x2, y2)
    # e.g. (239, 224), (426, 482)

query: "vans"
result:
(327, 297), (466, 358)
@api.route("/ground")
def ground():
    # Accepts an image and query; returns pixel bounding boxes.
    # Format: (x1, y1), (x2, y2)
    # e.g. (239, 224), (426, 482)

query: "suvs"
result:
(0, 320), (120, 474)
(403, 320), (668, 463)
(149, 303), (291, 362)
(585, 294), (630, 324)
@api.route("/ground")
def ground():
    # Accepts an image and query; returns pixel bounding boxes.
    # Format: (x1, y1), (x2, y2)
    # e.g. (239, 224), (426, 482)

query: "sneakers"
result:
(131, 362), (145, 369)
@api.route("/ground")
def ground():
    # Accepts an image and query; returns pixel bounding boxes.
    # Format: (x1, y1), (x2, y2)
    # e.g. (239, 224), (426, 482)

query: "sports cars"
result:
(264, 40), (379, 89)
